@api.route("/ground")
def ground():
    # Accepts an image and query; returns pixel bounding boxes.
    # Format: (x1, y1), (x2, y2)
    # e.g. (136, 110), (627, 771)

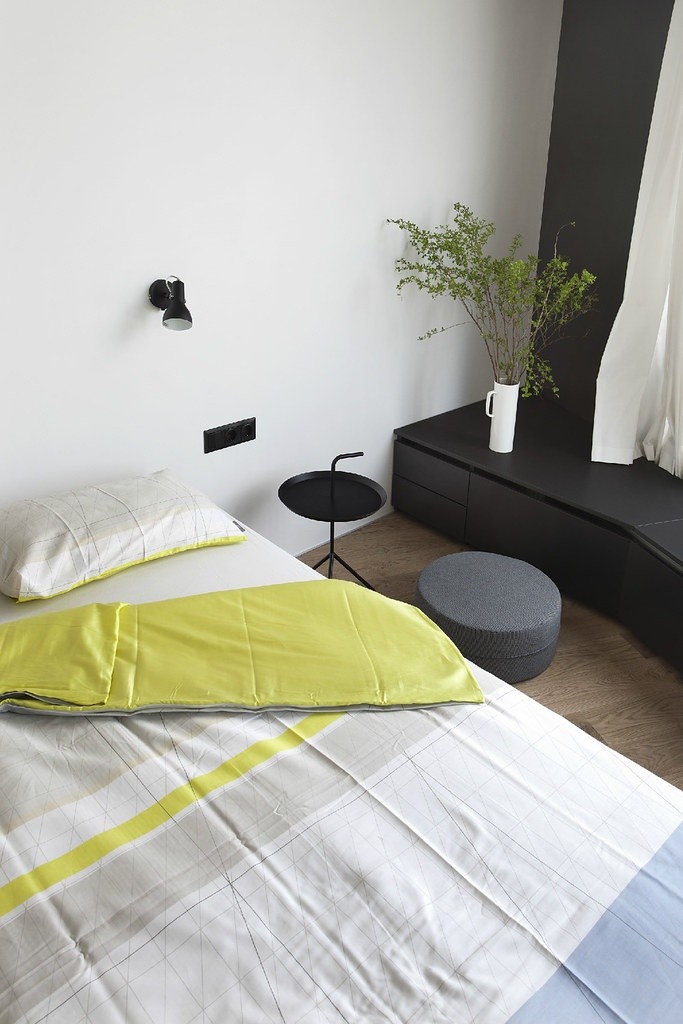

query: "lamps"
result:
(149, 275), (193, 331)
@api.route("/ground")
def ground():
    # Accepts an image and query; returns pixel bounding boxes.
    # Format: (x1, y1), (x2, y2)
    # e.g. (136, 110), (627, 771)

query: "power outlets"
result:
(203, 417), (256, 453)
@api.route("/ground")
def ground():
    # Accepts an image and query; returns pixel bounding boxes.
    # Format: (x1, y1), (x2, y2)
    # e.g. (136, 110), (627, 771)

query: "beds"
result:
(0, 509), (683, 1024)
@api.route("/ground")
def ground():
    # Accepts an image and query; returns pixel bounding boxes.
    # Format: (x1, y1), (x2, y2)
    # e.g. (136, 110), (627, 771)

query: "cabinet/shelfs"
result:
(390, 387), (683, 672)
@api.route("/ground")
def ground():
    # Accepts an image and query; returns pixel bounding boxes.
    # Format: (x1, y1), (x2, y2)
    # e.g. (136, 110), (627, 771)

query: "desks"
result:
(279, 451), (387, 593)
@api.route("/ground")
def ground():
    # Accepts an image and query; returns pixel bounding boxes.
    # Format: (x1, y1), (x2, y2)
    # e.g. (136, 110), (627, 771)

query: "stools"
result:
(417, 551), (562, 683)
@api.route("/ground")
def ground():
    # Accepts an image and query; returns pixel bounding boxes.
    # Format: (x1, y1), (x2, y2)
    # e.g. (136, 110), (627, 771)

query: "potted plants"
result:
(387, 203), (600, 454)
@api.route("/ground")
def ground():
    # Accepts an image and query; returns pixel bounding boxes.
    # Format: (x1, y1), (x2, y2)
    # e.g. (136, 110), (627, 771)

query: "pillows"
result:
(0, 470), (243, 602)
